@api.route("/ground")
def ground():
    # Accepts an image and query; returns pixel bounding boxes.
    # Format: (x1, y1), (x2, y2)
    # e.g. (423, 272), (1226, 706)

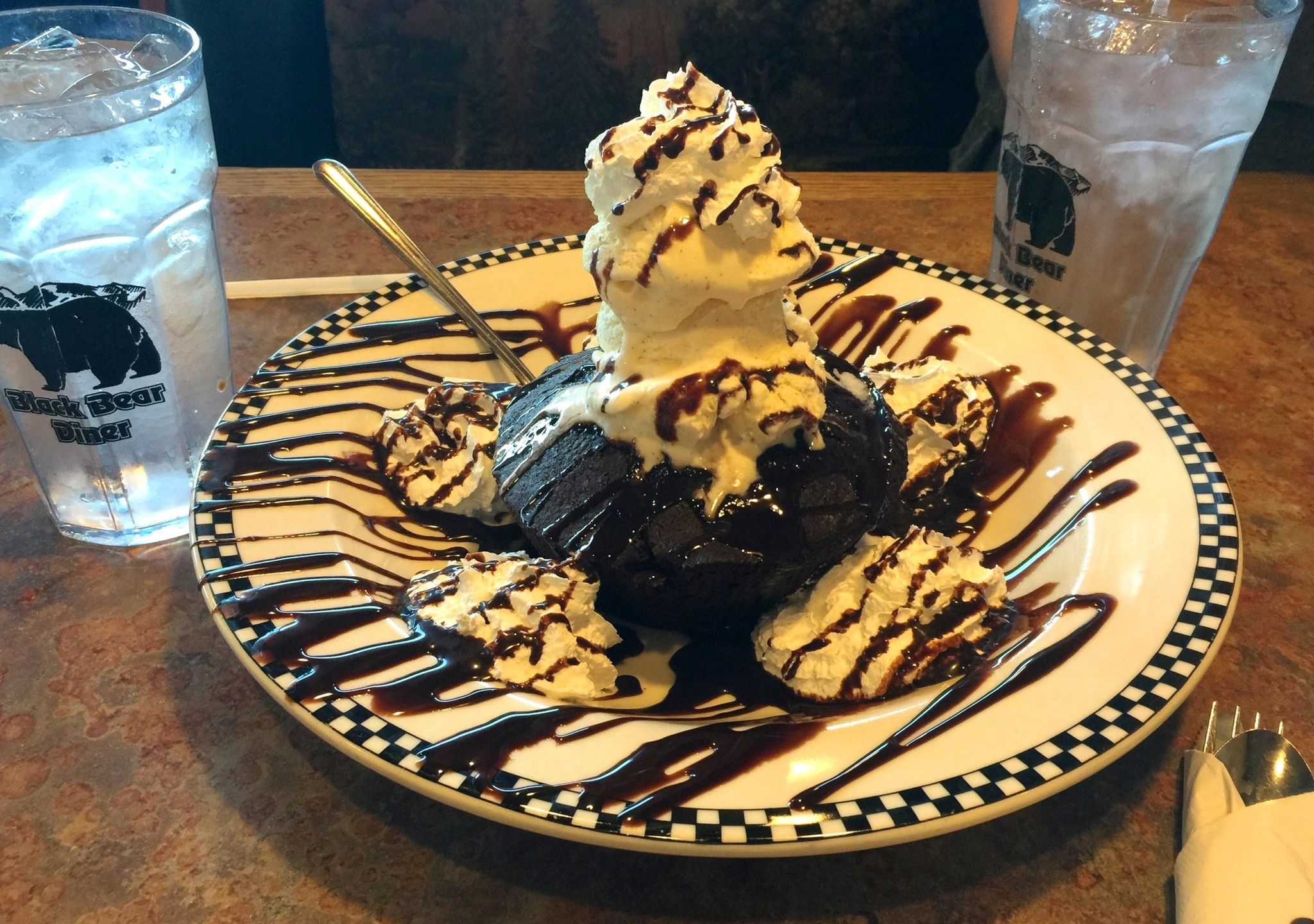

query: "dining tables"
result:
(0, 167), (1313, 923)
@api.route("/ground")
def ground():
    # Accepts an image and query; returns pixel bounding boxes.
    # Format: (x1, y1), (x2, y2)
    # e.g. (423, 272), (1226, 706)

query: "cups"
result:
(0, 6), (235, 545)
(984, 1), (1304, 378)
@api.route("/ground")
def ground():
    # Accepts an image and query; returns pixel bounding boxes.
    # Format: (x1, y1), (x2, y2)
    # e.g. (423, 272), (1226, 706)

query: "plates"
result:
(186, 238), (1247, 858)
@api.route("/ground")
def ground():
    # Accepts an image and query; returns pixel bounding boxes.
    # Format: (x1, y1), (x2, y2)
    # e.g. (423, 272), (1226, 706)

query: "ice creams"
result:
(363, 55), (1078, 718)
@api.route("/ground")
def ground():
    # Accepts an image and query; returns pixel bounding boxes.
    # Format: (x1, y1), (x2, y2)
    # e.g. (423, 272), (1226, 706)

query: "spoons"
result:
(1190, 703), (1314, 810)
